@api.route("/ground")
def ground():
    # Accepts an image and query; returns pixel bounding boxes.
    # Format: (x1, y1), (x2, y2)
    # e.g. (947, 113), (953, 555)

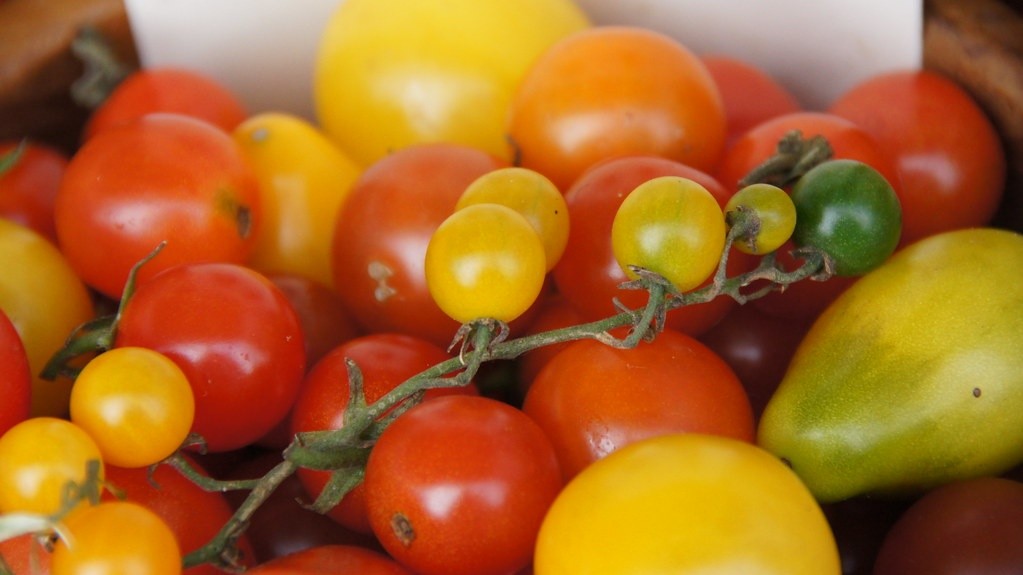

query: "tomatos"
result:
(0, 0), (1023, 575)
